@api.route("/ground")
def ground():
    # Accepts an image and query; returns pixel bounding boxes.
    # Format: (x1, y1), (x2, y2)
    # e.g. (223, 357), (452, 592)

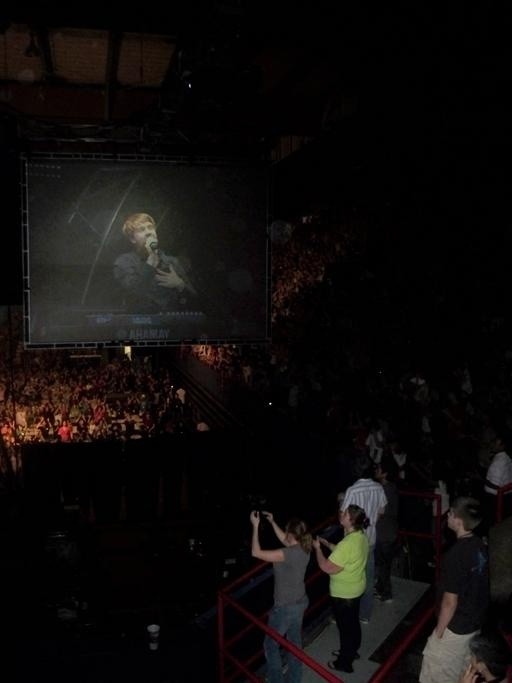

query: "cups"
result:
(148, 624), (161, 651)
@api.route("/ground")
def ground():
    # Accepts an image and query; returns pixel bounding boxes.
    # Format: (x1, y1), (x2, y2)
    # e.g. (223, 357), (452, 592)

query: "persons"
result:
(337, 454), (389, 619)
(458, 630), (512, 682)
(307, 300), (512, 548)
(0, 340), (208, 474)
(312, 503), (372, 672)
(180, 229), (345, 393)
(249, 508), (314, 682)
(105, 212), (197, 311)
(373, 459), (401, 599)
(417, 496), (489, 683)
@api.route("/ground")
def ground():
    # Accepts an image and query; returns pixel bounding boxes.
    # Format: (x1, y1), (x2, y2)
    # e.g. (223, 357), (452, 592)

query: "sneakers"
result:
(330, 647), (361, 659)
(328, 615), (337, 625)
(371, 591), (394, 604)
(327, 660), (354, 673)
(359, 616), (369, 624)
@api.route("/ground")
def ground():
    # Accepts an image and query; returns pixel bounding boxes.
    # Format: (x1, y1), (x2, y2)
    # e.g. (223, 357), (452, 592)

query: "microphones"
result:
(150, 243), (158, 250)
